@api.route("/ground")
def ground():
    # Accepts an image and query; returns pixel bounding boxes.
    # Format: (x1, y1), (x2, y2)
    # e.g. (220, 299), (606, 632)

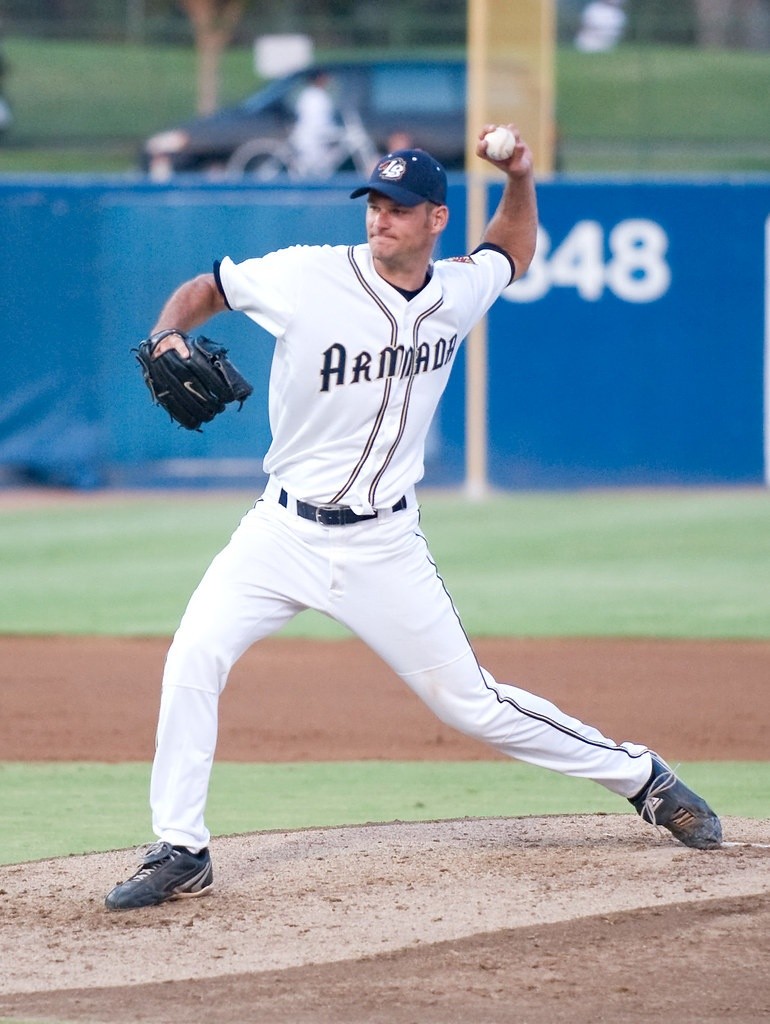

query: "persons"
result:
(287, 67), (340, 176)
(105, 122), (725, 913)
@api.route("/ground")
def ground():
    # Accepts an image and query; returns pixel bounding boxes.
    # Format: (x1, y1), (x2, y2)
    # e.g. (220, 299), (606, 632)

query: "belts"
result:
(278, 488), (407, 531)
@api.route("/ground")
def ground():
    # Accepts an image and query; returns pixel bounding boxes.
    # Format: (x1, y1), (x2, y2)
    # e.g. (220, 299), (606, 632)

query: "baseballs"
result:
(483, 128), (515, 161)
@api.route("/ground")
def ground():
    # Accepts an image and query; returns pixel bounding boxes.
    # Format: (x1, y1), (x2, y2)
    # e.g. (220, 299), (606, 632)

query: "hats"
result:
(349, 149), (446, 207)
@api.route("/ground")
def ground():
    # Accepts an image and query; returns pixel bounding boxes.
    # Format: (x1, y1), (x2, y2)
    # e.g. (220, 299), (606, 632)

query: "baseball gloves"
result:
(129, 328), (255, 434)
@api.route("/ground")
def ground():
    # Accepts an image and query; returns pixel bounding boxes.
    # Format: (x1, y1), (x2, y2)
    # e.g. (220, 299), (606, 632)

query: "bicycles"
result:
(227, 108), (382, 178)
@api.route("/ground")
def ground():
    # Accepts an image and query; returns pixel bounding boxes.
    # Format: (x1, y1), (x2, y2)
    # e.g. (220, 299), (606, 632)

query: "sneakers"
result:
(105, 840), (214, 912)
(630, 749), (722, 850)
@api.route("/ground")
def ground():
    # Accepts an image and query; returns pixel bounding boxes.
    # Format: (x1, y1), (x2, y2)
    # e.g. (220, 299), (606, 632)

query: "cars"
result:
(131, 58), (469, 177)
